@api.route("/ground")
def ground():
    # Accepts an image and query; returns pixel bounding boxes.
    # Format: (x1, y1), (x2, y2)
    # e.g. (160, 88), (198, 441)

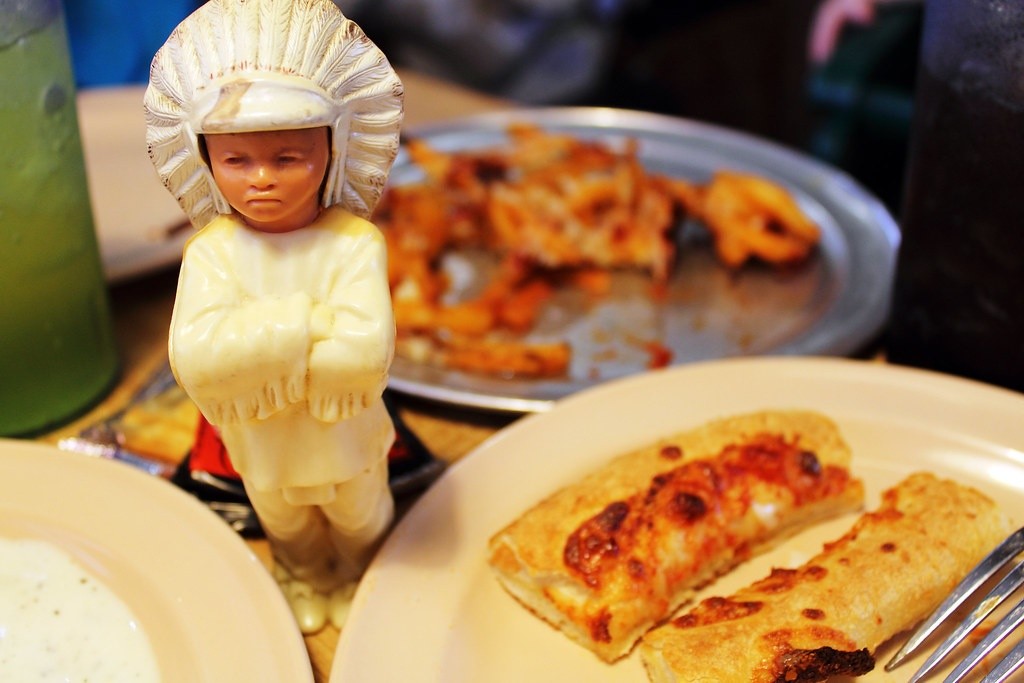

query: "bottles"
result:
(0, 1), (124, 441)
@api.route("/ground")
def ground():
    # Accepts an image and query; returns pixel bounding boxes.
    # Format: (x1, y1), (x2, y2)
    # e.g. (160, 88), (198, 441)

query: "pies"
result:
(483, 410), (869, 661)
(641, 471), (1015, 683)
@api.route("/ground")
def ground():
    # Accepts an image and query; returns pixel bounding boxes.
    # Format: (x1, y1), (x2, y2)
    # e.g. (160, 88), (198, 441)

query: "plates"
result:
(76, 86), (197, 280)
(386, 109), (902, 412)
(331, 356), (1024, 683)
(2, 438), (315, 683)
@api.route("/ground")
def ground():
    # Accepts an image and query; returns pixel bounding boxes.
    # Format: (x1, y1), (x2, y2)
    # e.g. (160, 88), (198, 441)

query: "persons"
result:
(595, 0), (1024, 395)
(142, 0), (402, 631)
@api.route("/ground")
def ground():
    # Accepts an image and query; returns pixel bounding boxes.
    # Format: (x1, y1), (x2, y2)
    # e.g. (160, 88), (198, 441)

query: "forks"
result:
(883, 527), (1024, 682)
